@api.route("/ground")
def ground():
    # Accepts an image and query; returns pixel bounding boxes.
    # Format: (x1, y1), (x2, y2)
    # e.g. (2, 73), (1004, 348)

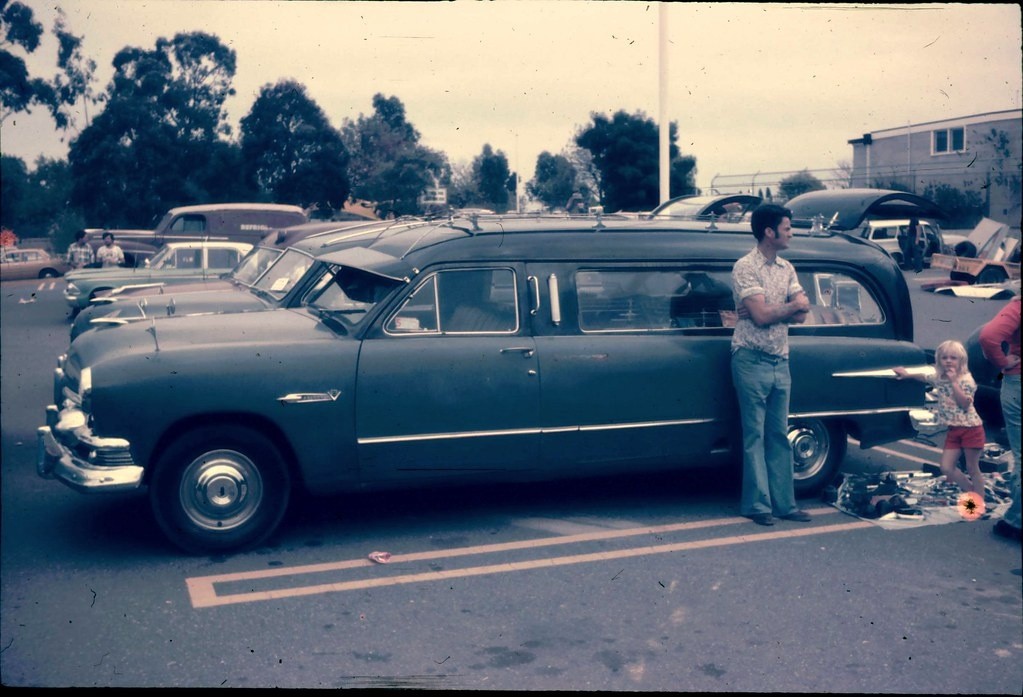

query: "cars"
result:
(1, 249), (66, 280)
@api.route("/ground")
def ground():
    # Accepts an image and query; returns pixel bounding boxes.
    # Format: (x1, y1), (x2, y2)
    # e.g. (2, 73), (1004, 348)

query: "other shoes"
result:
(750, 513), (777, 527)
(776, 508), (812, 524)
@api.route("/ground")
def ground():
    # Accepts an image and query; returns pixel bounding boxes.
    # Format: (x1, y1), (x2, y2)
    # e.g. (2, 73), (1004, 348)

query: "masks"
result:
(1009, 279), (1022, 298)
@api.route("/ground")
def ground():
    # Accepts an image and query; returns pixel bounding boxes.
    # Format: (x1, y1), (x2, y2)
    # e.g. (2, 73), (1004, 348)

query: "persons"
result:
(728, 204), (813, 526)
(901, 218), (923, 274)
(96, 231), (128, 270)
(979, 275), (1021, 536)
(66, 229), (96, 319)
(892, 340), (989, 521)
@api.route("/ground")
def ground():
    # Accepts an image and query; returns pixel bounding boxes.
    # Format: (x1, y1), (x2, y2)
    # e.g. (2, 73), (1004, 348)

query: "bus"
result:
(32, 180), (953, 545)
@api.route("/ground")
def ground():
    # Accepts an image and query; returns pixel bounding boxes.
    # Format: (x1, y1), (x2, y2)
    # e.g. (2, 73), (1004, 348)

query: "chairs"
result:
(442, 297), (518, 332)
